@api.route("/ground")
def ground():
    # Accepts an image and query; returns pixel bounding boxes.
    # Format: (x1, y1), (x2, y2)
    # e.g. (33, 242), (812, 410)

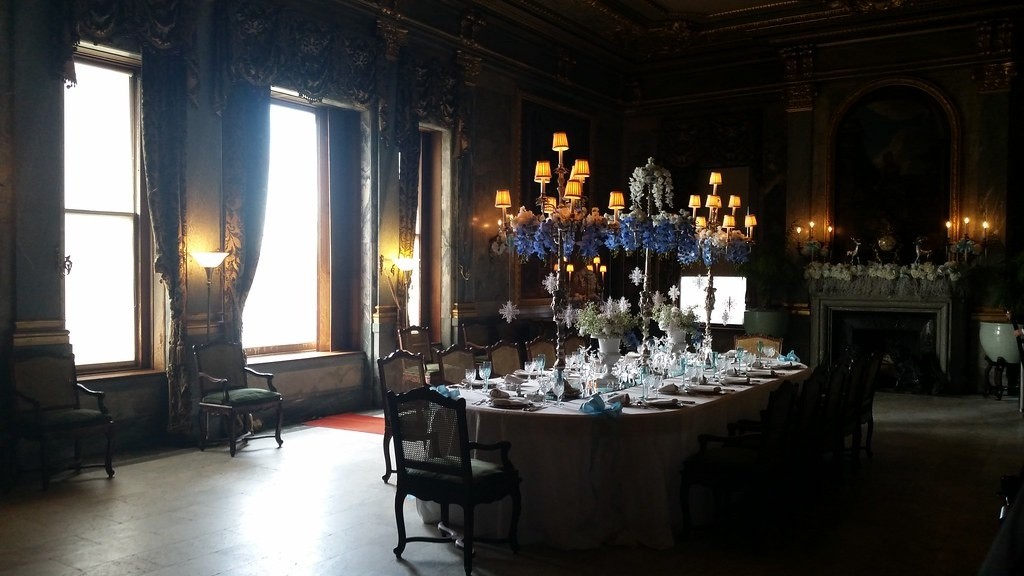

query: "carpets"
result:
(302, 413), (389, 432)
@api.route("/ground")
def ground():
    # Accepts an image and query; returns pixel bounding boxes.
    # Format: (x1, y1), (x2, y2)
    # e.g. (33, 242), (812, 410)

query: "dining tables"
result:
(434, 349), (814, 553)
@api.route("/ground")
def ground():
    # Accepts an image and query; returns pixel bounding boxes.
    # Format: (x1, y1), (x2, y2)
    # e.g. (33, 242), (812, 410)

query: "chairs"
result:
(376, 323), (585, 482)
(190, 337), (284, 458)
(384, 384), (521, 576)
(681, 340), (891, 557)
(4, 344), (114, 490)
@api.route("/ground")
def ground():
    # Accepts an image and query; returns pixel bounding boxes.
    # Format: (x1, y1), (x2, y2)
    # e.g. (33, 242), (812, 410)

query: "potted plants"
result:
(651, 304), (696, 358)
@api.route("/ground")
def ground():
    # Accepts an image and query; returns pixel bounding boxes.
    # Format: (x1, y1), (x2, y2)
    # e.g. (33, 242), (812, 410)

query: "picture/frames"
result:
(514, 90), (597, 308)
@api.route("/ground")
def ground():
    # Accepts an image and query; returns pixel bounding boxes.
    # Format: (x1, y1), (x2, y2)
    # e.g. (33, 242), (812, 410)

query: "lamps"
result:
(190, 252), (230, 343)
(493, 133), (625, 388)
(393, 257), (422, 327)
(685, 172), (759, 368)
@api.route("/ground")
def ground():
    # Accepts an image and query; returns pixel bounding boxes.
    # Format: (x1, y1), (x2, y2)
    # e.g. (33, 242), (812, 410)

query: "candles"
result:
(946, 216), (989, 243)
(796, 221), (832, 245)
(575, 295), (641, 387)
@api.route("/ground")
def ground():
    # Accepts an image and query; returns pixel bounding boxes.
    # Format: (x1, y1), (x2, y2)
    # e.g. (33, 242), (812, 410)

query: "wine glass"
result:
(465, 341), (776, 409)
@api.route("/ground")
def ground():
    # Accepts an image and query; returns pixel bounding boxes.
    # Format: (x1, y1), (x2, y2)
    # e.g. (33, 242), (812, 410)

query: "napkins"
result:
(459, 346), (791, 407)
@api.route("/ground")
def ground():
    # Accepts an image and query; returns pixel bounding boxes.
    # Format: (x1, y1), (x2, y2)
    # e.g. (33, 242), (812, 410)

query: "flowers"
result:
(508, 156), (749, 266)
(804, 260), (961, 283)
